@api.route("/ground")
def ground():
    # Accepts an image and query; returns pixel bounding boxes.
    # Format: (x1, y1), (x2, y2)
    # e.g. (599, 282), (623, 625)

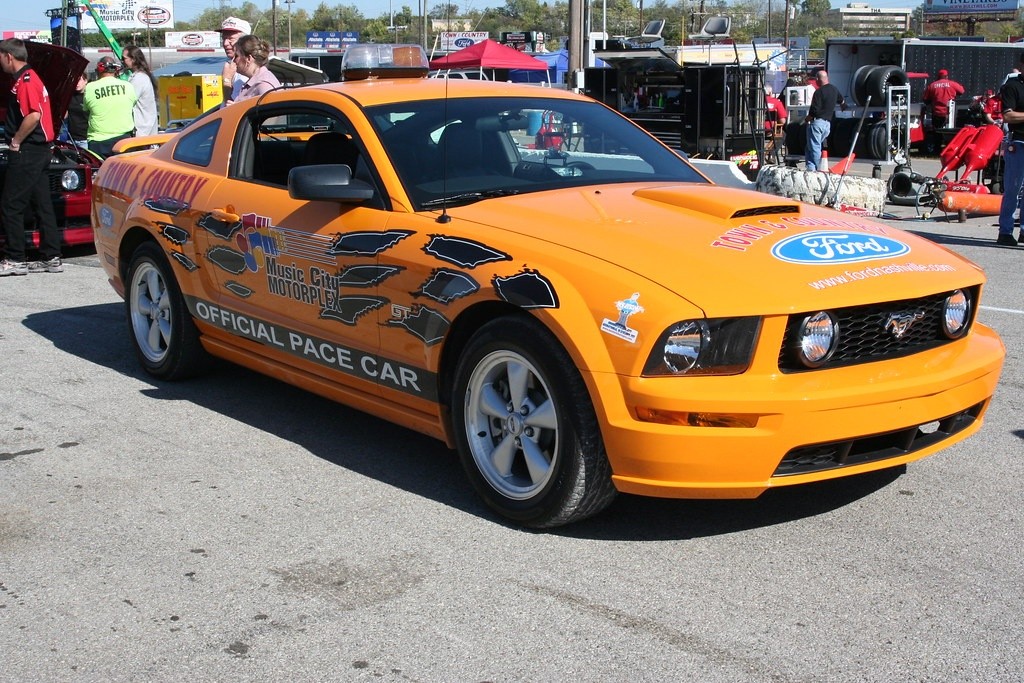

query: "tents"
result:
(429, 38), (551, 88)
(511, 49), (613, 153)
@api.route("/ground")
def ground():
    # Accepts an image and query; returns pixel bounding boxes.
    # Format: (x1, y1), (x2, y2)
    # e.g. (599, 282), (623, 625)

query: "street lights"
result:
(284, 0), (298, 61)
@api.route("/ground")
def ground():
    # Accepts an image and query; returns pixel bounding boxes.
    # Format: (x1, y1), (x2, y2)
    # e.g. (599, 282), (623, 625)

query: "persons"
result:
(799, 70), (847, 171)
(977, 51), (1024, 246)
(214, 16), (283, 110)
(64, 44), (159, 160)
(922, 69), (966, 144)
(0, 38), (64, 276)
(763, 82), (788, 164)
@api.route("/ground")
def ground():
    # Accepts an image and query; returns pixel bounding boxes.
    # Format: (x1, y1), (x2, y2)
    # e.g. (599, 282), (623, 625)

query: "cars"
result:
(1, 36), (104, 260)
(91, 43), (1007, 532)
(424, 69), (490, 82)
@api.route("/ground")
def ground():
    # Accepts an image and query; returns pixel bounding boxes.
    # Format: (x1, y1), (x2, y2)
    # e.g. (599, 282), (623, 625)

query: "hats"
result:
(214, 17), (252, 36)
(938, 69), (949, 76)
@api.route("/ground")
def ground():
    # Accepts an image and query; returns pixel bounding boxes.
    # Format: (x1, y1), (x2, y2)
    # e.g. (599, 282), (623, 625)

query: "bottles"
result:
(659, 92), (663, 107)
(633, 92), (639, 112)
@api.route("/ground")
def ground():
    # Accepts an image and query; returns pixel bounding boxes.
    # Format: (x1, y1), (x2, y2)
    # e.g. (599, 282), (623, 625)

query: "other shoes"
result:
(997, 233), (1018, 246)
(1017, 233), (1024, 243)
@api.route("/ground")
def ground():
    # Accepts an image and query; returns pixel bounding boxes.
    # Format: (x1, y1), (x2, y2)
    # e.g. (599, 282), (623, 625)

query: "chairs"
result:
(765, 108), (785, 156)
(434, 123), (499, 185)
(300, 130), (353, 164)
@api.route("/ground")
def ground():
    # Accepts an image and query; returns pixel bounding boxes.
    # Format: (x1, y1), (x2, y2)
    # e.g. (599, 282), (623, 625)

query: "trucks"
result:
(582, 36), (1024, 165)
(81, 47), (346, 87)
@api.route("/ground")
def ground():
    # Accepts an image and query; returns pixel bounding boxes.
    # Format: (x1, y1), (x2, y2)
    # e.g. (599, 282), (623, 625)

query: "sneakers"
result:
(0, 257), (28, 276)
(27, 257), (65, 272)
